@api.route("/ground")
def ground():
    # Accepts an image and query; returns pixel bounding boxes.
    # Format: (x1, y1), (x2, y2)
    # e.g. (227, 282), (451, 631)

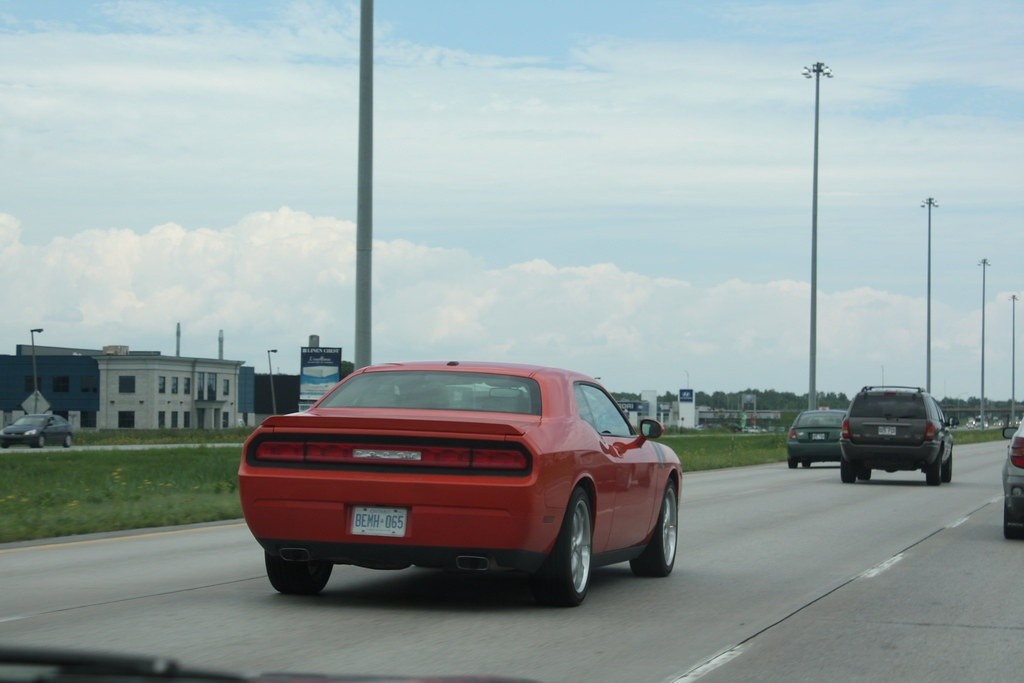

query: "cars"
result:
(787, 410), (847, 468)
(1003, 416), (1024, 539)
(238, 362), (683, 607)
(0, 414), (74, 448)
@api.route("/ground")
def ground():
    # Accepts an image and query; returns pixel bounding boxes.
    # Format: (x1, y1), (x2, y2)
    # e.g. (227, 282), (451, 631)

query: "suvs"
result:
(840, 386), (953, 486)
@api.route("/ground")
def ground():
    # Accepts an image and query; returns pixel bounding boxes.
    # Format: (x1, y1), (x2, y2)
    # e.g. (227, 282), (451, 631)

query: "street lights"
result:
(268, 350), (277, 415)
(977, 257), (991, 429)
(802, 61), (832, 410)
(31, 329), (43, 414)
(921, 198), (938, 393)
(1009, 295), (1018, 427)
